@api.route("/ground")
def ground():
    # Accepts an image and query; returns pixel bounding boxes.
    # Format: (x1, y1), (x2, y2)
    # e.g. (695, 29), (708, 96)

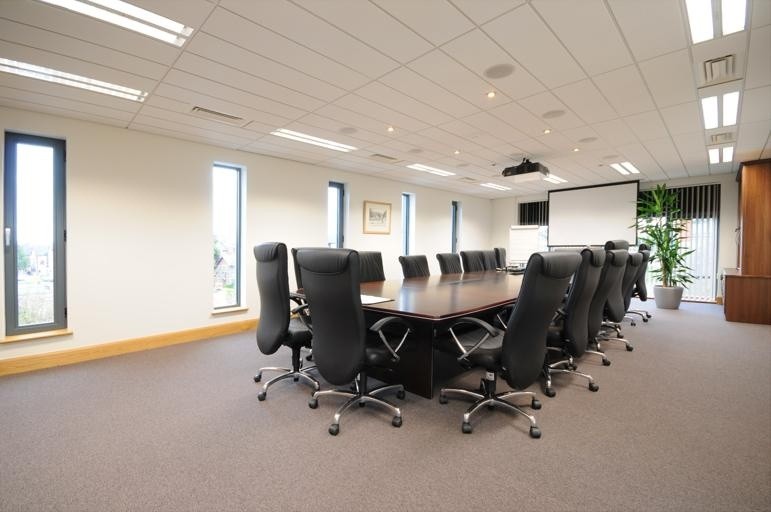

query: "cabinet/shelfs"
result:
(719, 158), (771, 326)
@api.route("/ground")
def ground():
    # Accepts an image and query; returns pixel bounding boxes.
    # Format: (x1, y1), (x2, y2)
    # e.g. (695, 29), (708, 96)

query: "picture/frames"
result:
(363, 200), (392, 235)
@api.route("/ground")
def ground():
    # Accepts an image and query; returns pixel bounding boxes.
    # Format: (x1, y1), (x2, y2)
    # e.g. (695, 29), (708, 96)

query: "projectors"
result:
(502, 159), (551, 185)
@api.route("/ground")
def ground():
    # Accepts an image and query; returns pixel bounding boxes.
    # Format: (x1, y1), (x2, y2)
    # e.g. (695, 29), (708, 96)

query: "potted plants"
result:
(627, 183), (698, 309)
